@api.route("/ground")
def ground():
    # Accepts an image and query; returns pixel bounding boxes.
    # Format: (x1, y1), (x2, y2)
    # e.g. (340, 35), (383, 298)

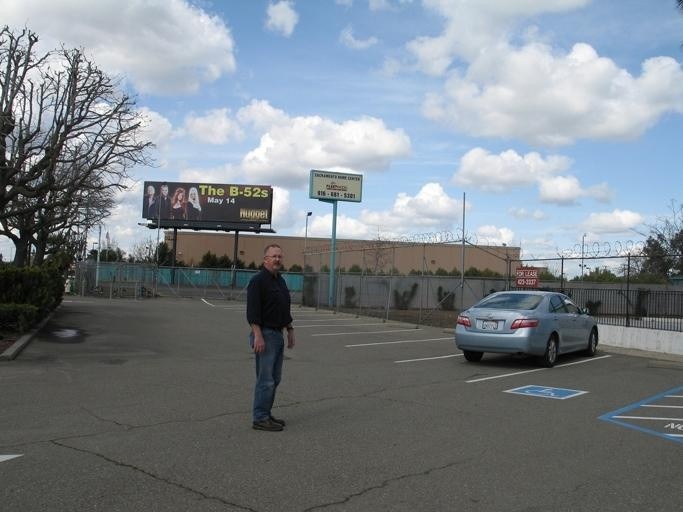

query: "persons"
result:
(63, 278), (71, 296)
(143, 185), (155, 219)
(153, 184), (171, 221)
(185, 187), (202, 220)
(245, 243), (294, 432)
(168, 187), (188, 220)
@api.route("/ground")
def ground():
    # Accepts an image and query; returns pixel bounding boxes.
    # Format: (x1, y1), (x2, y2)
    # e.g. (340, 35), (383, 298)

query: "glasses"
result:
(264, 254), (282, 259)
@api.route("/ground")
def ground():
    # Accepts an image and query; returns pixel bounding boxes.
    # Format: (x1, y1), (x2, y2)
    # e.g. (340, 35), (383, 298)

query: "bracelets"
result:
(287, 325), (294, 331)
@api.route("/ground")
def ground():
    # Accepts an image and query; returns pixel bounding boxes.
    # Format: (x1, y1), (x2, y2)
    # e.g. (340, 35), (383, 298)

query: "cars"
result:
(453, 290), (599, 366)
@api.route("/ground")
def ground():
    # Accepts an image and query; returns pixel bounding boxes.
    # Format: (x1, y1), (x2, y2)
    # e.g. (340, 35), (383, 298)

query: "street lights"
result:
(303, 211), (312, 248)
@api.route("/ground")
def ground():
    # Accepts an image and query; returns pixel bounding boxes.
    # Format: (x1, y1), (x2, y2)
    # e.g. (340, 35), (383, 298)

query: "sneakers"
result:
(251, 414), (285, 433)
